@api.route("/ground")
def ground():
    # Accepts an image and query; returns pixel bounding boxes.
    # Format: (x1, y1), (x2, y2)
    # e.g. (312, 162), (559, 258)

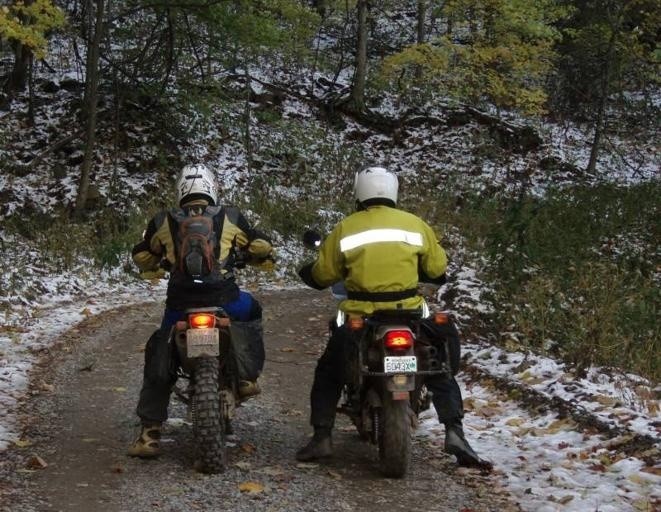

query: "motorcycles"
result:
(141, 229), (276, 473)
(302, 230), (453, 478)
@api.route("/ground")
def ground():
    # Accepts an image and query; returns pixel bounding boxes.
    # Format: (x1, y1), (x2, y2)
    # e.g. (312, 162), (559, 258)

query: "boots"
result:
(296, 426), (336, 461)
(444, 420), (488, 468)
(128, 421), (162, 456)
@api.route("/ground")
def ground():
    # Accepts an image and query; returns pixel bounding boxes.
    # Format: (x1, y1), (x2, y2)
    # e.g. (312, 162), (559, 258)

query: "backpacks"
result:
(167, 206), (225, 288)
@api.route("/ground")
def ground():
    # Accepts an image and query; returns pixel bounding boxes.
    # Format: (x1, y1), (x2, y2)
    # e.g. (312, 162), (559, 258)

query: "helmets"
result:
(354, 166), (399, 207)
(176, 163), (218, 207)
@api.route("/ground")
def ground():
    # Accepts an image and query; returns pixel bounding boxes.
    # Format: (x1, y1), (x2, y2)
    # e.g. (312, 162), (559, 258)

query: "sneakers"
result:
(238, 376), (261, 396)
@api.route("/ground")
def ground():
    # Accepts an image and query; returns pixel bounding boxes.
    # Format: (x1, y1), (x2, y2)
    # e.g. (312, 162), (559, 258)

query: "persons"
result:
(294, 166), (493, 473)
(128, 162), (274, 460)
